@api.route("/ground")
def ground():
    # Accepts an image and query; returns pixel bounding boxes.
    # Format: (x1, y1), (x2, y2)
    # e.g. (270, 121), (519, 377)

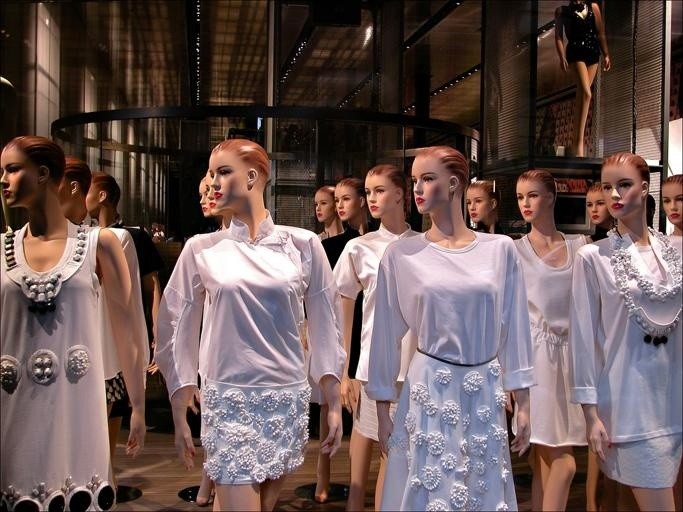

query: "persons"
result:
(154, 140), (347, 510)
(332, 147), (683, 511)
(556, 1), (611, 157)
(1, 134), (165, 511)
